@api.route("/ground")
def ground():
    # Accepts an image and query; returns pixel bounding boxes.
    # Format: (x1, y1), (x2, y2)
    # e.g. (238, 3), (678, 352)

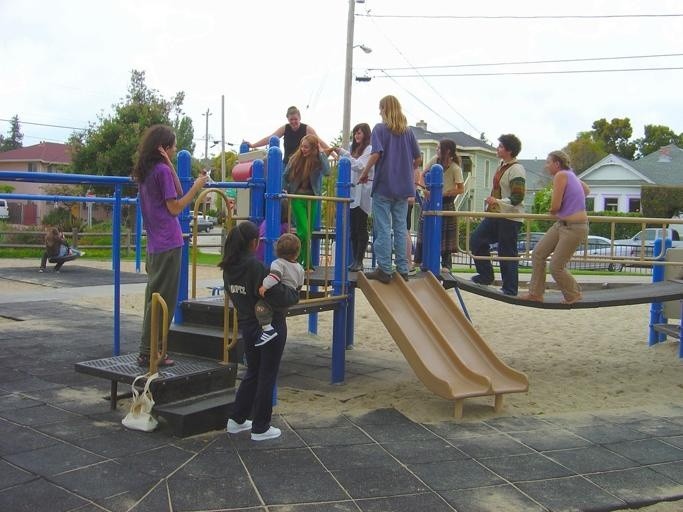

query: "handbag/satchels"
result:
(121, 370), (159, 433)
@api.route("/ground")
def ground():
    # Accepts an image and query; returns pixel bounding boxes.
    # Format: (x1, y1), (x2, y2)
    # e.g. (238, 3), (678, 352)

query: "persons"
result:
(132, 125), (209, 367)
(39, 231), (66, 273)
(517, 151), (590, 304)
(218, 222), (300, 441)
(45, 228), (86, 259)
(255, 233), (306, 347)
(470, 134), (526, 296)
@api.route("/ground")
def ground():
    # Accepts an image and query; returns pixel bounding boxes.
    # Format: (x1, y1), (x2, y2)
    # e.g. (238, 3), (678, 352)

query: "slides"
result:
(356, 269), (529, 399)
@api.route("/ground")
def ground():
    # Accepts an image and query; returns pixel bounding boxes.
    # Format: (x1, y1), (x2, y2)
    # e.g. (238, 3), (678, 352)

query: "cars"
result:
(485, 231), (552, 259)
(0, 199), (9, 218)
(187, 209), (216, 234)
(516, 234), (638, 276)
(365, 229), (418, 254)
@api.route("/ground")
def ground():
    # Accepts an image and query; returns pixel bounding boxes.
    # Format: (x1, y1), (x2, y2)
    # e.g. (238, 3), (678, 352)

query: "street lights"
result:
(201, 108), (214, 175)
(212, 139), (235, 182)
(341, 43), (377, 161)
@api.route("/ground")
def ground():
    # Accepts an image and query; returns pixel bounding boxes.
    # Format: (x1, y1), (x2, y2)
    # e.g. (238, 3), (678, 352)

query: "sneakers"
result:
(367, 269), (390, 283)
(226, 418), (252, 434)
(250, 426), (282, 442)
(255, 329), (280, 347)
(138, 354), (175, 368)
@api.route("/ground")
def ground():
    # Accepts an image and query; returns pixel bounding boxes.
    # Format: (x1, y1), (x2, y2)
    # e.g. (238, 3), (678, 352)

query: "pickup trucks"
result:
(607, 228), (683, 268)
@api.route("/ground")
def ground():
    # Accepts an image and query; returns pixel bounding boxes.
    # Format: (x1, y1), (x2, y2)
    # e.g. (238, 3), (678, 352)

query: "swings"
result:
(49, 200), (80, 263)
